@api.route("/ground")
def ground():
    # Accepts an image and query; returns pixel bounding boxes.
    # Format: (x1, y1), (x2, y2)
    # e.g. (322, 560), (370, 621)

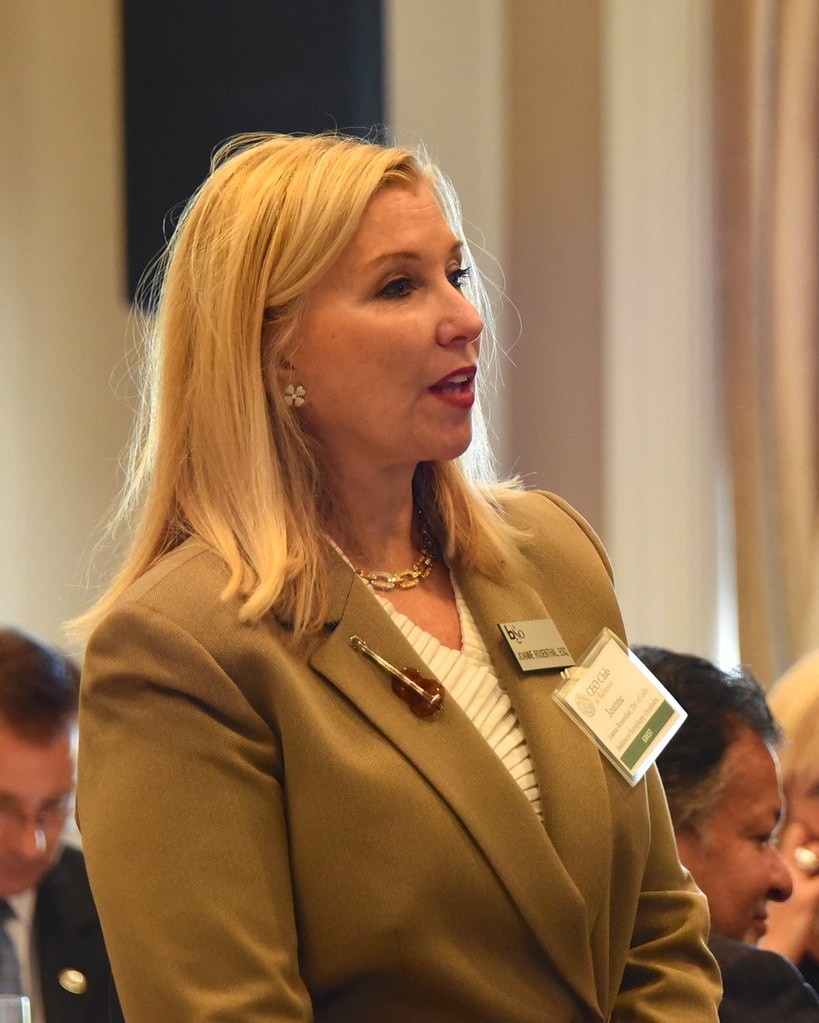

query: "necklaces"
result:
(284, 488), (452, 592)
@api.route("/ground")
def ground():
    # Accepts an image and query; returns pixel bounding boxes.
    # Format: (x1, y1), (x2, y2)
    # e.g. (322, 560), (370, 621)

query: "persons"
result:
(610, 644), (819, 1023)
(757, 649), (819, 1003)
(73, 125), (722, 1023)
(0, 627), (132, 1023)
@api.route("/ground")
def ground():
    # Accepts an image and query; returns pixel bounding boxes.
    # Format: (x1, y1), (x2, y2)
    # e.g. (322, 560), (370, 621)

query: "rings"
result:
(791, 840), (818, 878)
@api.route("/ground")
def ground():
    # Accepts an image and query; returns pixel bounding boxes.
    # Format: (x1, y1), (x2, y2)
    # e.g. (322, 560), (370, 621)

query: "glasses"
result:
(7, 805), (72, 834)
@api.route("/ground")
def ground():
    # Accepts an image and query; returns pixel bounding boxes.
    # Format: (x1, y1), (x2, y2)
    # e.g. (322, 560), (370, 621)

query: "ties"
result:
(1, 899), (22, 997)
